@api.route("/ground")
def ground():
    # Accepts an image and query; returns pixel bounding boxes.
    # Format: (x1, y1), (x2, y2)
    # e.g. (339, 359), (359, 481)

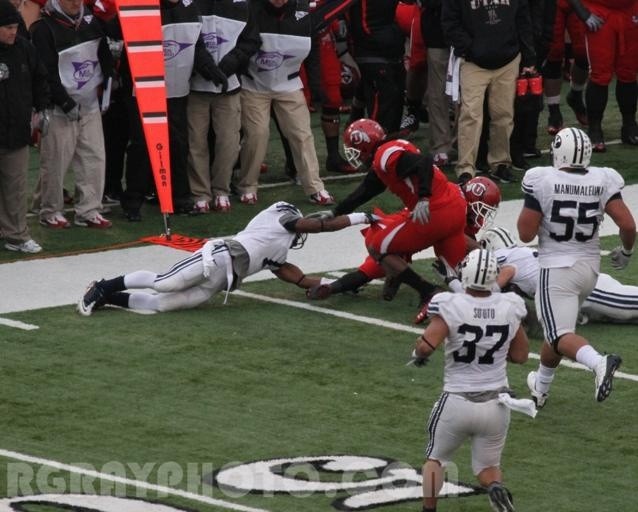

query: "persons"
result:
(407, 249), (529, 511)
(515, 125), (637, 410)
(0, 0), (638, 326)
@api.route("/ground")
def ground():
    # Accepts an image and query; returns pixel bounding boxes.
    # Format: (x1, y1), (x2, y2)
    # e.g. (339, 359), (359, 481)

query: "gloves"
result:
(63, 102), (81, 121)
(409, 200), (430, 224)
(208, 63), (227, 92)
(610, 246), (633, 269)
(305, 210), (333, 220)
(587, 14), (604, 30)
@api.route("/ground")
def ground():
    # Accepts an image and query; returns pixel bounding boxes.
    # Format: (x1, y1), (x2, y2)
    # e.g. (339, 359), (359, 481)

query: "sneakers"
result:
(77, 280), (104, 315)
(307, 284), (331, 300)
(526, 372), (548, 408)
(400, 116), (637, 182)
(489, 485), (514, 512)
(6, 194), (257, 252)
(594, 354), (621, 401)
(309, 191), (335, 205)
(414, 288), (442, 323)
(326, 158), (357, 173)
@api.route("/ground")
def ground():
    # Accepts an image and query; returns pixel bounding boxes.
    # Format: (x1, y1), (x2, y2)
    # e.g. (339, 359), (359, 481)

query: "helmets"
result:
(343, 118), (384, 170)
(551, 127), (593, 169)
(459, 248), (500, 292)
(480, 226), (516, 252)
(464, 176), (501, 227)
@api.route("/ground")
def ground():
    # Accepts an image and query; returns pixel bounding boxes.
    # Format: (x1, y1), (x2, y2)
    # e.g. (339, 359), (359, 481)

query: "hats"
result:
(0, 0), (20, 26)
